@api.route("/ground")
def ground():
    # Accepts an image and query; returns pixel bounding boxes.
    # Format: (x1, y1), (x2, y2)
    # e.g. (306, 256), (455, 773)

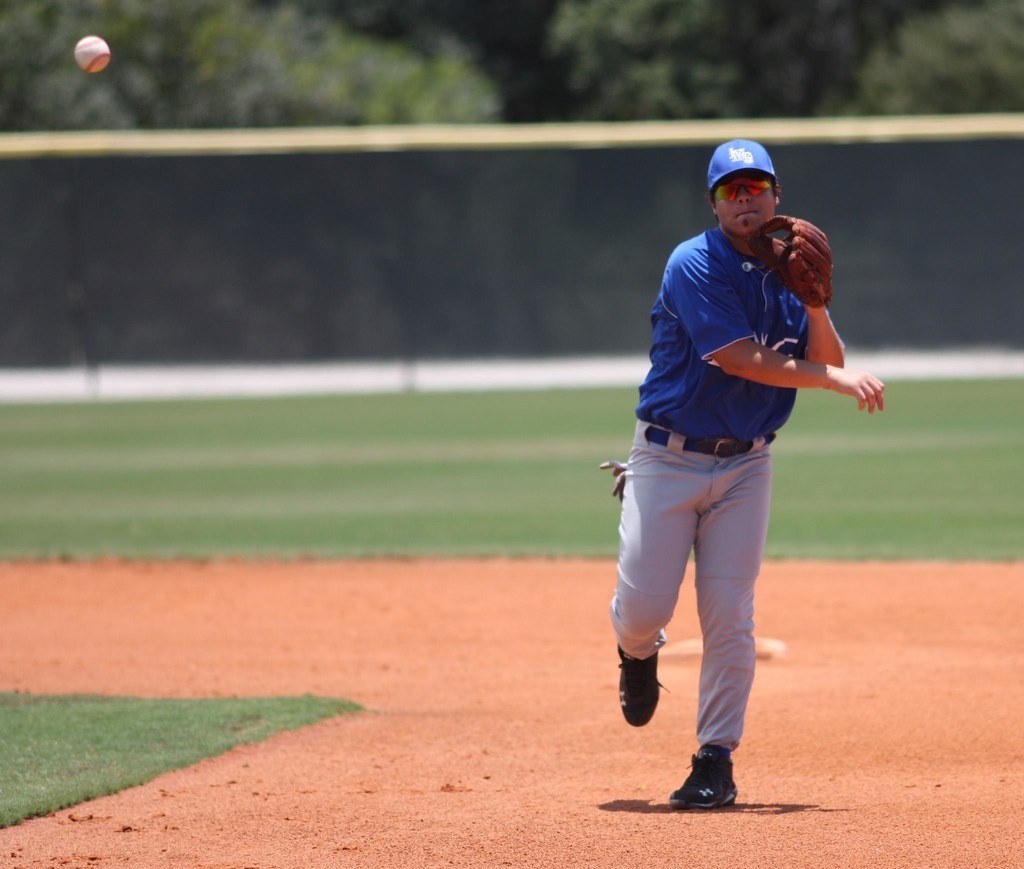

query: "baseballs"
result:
(74, 35), (111, 74)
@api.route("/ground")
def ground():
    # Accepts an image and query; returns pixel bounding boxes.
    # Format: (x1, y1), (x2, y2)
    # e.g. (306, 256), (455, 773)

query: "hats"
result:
(706, 138), (777, 192)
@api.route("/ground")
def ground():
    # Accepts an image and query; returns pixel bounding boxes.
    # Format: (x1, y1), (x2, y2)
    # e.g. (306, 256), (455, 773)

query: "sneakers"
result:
(618, 642), (671, 727)
(669, 743), (738, 808)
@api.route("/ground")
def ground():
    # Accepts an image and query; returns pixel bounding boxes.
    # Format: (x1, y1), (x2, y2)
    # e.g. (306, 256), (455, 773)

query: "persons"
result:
(611, 139), (885, 809)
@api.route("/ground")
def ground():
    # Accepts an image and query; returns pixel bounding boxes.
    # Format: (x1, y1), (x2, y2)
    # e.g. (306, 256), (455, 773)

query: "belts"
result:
(645, 425), (776, 458)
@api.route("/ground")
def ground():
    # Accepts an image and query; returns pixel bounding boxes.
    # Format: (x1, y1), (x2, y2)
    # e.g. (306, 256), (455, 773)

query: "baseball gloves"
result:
(745, 215), (833, 309)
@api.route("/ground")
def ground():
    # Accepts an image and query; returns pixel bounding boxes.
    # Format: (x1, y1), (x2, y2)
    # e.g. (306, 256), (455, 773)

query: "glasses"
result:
(712, 175), (775, 202)
